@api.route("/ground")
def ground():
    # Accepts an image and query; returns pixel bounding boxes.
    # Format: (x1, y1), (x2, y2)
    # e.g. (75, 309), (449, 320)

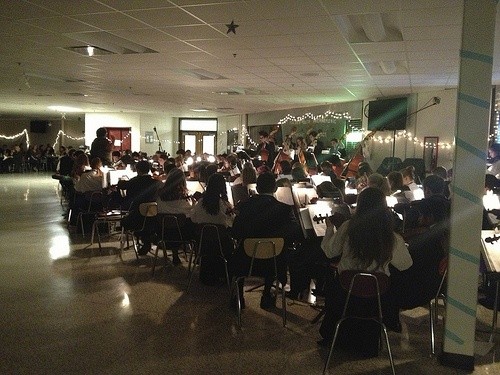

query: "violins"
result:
(180, 178), (420, 234)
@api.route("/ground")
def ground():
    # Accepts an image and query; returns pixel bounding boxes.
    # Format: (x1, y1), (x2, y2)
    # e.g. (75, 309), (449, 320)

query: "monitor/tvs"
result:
(368, 97), (407, 130)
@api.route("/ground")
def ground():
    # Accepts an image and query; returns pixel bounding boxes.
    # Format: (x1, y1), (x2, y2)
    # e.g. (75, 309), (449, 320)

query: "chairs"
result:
(151, 213), (193, 279)
(232, 238), (288, 328)
(190, 222), (231, 294)
(322, 270), (396, 375)
(51, 171), (136, 257)
(410, 256), (448, 356)
(118, 202), (157, 259)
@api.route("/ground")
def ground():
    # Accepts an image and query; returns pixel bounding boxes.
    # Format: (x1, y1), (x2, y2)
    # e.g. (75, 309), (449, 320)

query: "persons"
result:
(1, 126), (500, 332)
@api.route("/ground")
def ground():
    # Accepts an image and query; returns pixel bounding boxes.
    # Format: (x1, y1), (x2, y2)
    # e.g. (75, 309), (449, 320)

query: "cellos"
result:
(255, 125), (279, 162)
(271, 126), (298, 175)
(294, 129), (325, 165)
(335, 128), (377, 179)
